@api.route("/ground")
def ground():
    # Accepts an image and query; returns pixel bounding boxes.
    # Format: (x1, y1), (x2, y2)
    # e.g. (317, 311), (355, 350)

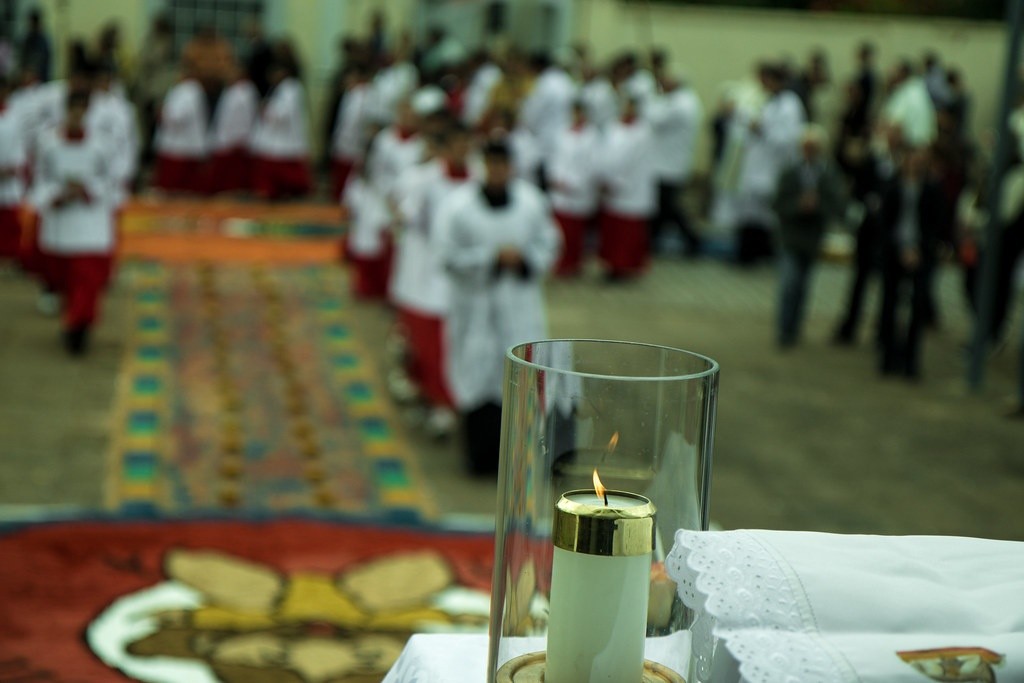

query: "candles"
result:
(549, 429), (656, 495)
(542, 467), (658, 683)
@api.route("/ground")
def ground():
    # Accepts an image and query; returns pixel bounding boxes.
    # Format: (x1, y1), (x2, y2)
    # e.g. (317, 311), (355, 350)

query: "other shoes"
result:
(427, 405), (460, 439)
(65, 320), (90, 357)
(387, 367), (418, 405)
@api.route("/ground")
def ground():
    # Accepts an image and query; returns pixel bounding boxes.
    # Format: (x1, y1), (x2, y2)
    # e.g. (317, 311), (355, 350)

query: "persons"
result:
(338, 89), (583, 485)
(0, 7), (712, 285)
(697, 39), (1024, 421)
(37, 82), (124, 353)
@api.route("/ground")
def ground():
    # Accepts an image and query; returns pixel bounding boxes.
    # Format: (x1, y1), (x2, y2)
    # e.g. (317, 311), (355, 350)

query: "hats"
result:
(798, 123), (829, 155)
